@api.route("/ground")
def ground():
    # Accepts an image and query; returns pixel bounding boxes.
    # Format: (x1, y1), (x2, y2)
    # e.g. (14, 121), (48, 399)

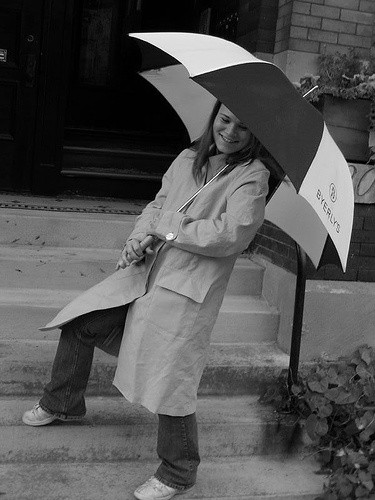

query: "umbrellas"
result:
(125, 31), (356, 274)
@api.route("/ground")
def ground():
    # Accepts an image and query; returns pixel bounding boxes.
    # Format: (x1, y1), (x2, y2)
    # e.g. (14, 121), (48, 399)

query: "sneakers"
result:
(133, 474), (196, 500)
(21, 401), (83, 427)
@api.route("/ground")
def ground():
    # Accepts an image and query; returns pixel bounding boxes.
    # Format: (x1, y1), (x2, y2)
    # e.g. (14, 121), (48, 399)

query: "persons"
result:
(23, 99), (270, 500)
